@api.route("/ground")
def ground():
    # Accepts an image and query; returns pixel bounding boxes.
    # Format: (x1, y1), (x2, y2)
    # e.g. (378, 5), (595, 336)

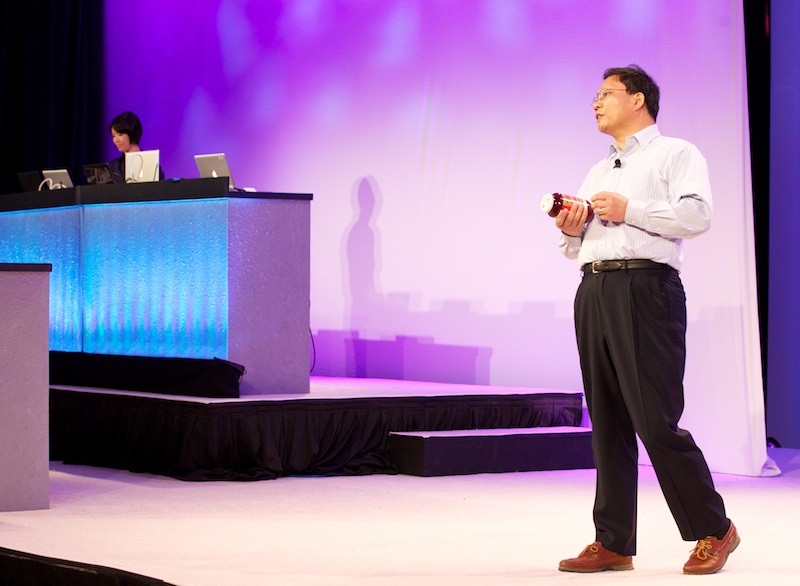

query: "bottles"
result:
(540, 192), (594, 224)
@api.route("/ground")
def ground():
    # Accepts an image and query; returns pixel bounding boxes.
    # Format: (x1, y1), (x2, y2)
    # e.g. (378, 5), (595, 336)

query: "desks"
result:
(0, 176), (312, 512)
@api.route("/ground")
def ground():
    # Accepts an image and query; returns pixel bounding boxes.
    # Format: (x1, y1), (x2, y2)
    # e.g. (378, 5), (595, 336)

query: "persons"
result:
(555, 65), (741, 574)
(105, 111), (165, 182)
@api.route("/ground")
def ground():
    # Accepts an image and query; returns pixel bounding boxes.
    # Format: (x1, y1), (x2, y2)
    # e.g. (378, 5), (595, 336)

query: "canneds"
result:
(540, 192), (594, 223)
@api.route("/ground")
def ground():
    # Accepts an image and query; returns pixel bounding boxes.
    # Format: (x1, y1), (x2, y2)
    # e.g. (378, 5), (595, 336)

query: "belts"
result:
(576, 259), (672, 272)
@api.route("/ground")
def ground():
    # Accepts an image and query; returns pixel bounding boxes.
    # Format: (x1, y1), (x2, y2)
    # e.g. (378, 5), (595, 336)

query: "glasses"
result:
(589, 88), (629, 107)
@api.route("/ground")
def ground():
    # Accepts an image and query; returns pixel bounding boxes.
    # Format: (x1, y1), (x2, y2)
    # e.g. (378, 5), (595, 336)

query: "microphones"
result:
(613, 159), (622, 170)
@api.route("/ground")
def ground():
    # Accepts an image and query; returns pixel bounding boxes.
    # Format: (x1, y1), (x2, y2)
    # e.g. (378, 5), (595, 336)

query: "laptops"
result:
(83, 163), (116, 185)
(124, 148), (160, 182)
(192, 153), (254, 191)
(40, 169), (72, 189)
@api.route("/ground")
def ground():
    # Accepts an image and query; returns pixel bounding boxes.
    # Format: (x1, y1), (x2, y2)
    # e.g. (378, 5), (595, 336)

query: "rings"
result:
(600, 208), (603, 215)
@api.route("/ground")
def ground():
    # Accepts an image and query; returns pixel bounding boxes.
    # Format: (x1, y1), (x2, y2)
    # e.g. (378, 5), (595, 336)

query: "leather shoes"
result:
(682, 518), (741, 574)
(558, 541), (634, 573)
